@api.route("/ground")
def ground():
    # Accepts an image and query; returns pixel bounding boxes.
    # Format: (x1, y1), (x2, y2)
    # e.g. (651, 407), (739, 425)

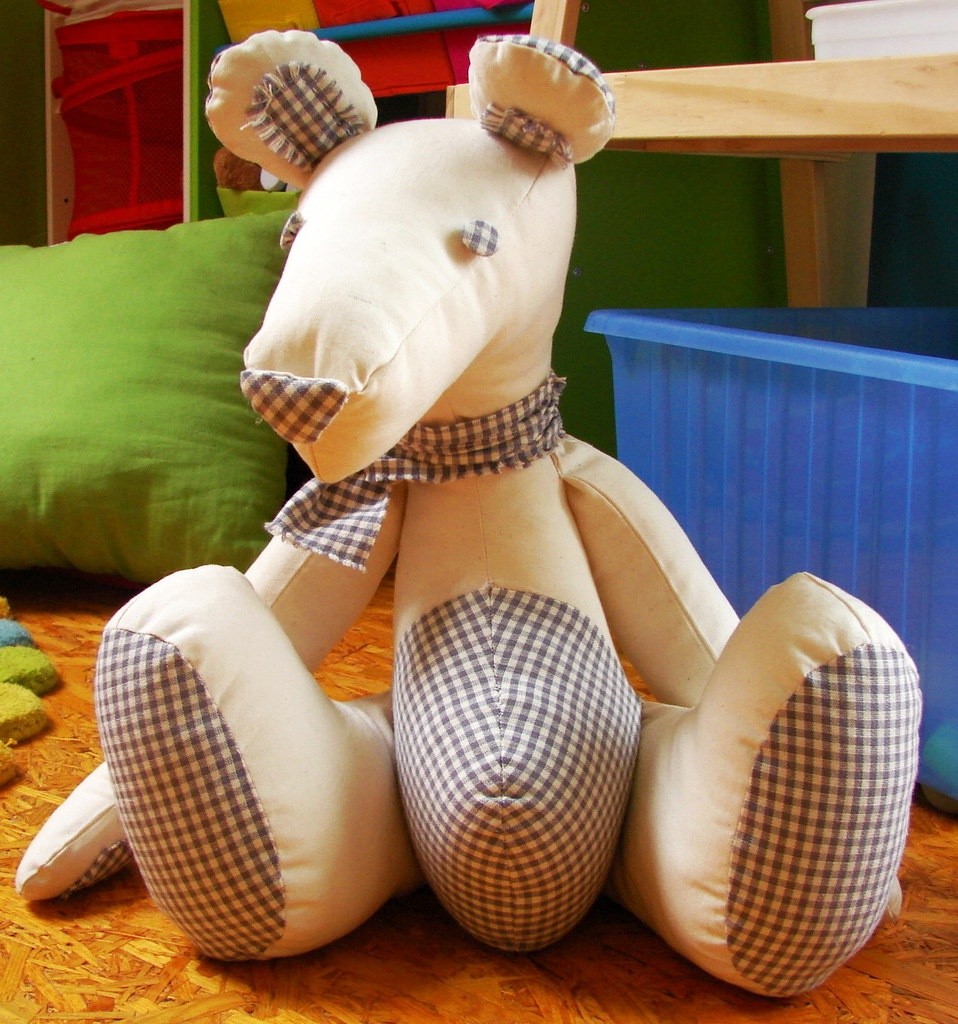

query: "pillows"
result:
(0, 206), (301, 586)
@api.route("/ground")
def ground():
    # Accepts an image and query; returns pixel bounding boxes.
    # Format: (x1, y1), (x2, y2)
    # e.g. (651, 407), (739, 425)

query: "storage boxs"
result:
(584, 306), (958, 801)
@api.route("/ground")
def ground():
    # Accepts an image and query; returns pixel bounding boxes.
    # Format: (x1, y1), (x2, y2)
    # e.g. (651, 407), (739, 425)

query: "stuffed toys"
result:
(13, 26), (930, 994)
